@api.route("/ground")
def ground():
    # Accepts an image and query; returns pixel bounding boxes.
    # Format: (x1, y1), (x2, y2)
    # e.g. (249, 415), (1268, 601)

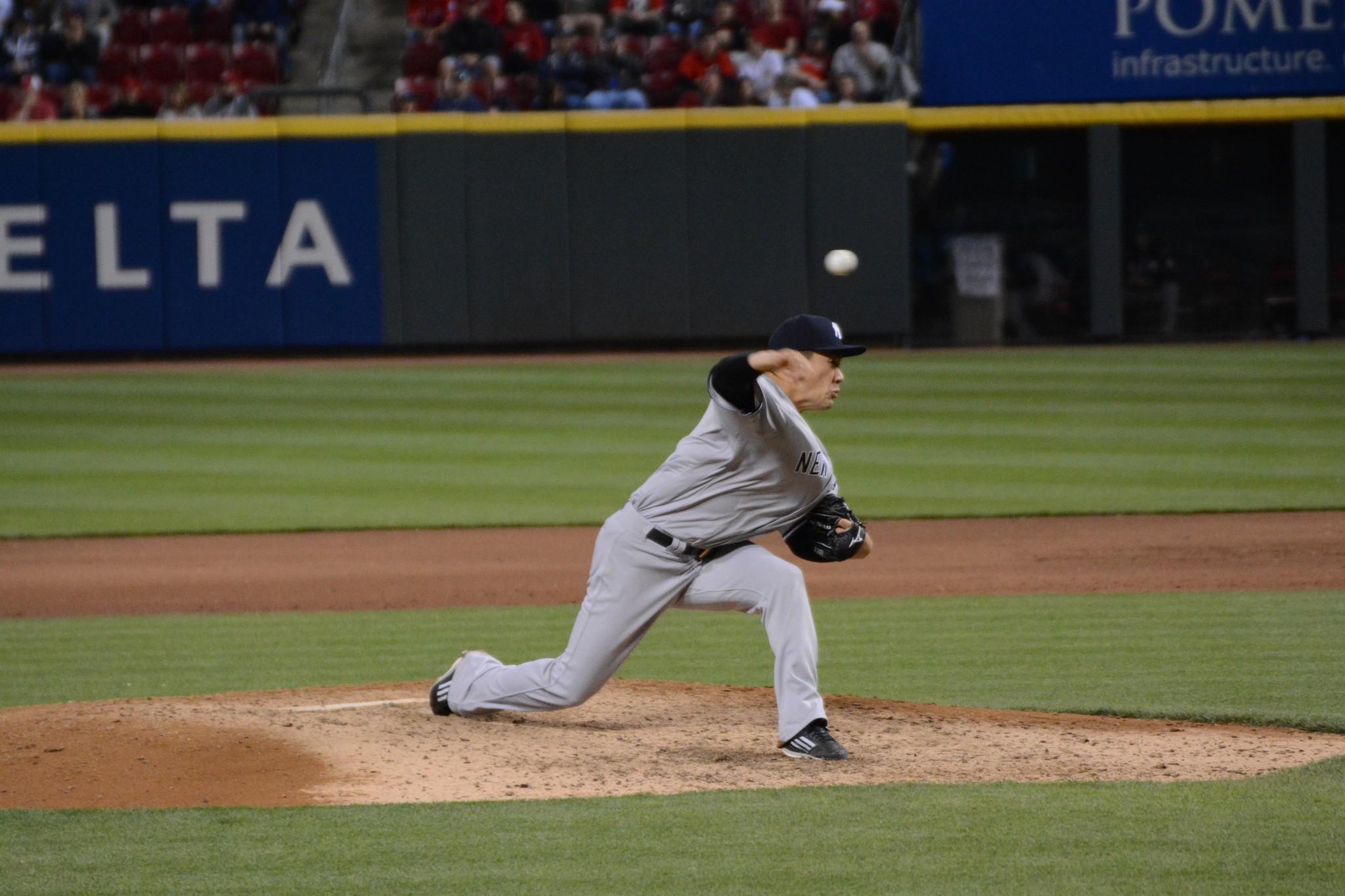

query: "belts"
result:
(648, 528), (710, 562)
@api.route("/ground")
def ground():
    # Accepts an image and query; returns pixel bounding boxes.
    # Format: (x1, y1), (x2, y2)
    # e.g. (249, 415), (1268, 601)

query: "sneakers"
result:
(782, 721), (849, 760)
(430, 649), (486, 715)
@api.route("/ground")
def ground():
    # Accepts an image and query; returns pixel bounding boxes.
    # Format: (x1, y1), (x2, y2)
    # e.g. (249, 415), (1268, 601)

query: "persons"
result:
(0, 0), (901, 126)
(430, 314), (873, 759)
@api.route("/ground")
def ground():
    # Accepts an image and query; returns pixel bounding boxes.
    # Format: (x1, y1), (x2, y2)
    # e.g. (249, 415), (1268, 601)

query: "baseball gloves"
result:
(789, 493), (867, 563)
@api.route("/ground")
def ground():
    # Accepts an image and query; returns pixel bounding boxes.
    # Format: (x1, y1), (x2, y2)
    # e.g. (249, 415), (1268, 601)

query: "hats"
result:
(767, 314), (865, 355)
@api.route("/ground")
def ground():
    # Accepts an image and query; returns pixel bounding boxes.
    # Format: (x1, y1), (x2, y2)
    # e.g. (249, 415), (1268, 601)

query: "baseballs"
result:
(823, 249), (860, 275)
